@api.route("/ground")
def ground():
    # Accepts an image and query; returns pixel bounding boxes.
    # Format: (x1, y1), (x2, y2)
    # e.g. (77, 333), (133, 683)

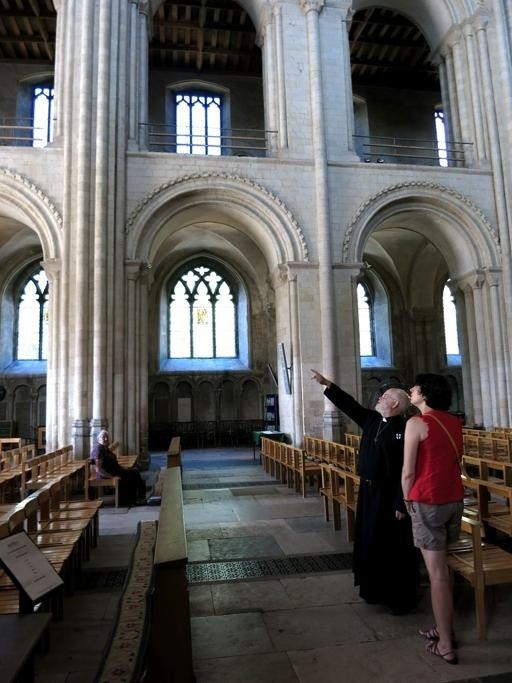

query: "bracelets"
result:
(403, 498), (413, 502)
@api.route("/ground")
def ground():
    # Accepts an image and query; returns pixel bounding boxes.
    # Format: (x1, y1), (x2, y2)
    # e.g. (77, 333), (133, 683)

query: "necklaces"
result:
(374, 420), (391, 442)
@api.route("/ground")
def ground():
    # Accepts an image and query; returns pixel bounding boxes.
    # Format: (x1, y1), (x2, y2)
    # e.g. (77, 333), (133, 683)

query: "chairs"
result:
(260, 424), (511, 644)
(1, 436), (140, 616)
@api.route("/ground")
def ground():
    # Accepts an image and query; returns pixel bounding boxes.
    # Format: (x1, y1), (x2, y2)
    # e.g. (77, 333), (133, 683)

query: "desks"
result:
(252, 431), (283, 464)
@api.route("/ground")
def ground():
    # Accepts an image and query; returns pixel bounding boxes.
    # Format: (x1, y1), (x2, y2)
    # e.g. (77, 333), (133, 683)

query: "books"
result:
(0, 531), (64, 601)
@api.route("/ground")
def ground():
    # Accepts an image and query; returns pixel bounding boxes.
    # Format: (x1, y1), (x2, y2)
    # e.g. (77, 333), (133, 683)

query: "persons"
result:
(92, 429), (146, 501)
(401, 373), (465, 665)
(310, 369), (420, 615)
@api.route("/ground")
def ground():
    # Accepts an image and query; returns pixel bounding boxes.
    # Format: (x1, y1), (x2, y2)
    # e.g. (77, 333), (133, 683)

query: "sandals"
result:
(417, 628), (458, 665)
